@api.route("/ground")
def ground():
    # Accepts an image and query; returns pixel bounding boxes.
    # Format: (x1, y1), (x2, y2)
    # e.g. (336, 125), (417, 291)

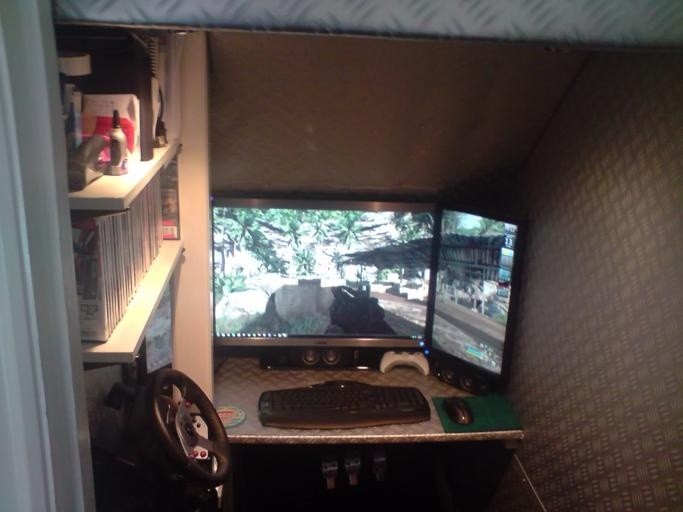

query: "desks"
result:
(213, 356), (525, 444)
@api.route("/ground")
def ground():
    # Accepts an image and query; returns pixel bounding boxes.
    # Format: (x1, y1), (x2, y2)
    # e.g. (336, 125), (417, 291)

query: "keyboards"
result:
(257, 379), (431, 428)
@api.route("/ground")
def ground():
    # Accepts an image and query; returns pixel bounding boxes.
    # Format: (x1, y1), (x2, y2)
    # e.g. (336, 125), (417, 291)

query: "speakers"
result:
(429, 359), (495, 397)
(260, 348), (356, 369)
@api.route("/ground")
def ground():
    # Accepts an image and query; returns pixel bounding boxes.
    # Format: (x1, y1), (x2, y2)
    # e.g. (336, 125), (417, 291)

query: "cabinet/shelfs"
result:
(66, 133), (184, 370)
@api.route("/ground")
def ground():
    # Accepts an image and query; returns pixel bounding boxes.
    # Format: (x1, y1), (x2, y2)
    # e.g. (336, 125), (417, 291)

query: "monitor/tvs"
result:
(213, 188), (435, 350)
(435, 199), (524, 382)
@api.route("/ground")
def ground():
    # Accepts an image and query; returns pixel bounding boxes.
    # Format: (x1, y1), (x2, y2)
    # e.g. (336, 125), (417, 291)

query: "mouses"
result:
(441, 397), (474, 424)
